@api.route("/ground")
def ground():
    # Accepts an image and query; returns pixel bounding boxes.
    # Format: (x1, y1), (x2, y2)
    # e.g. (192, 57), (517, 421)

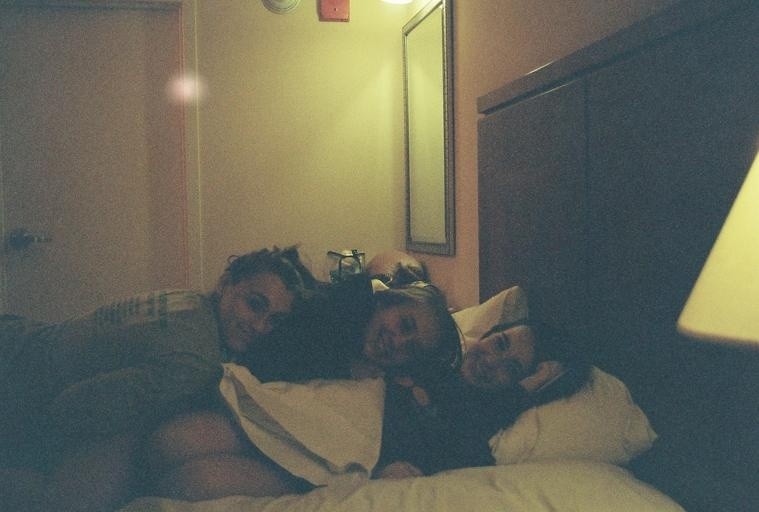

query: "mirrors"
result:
(399, 0), (457, 256)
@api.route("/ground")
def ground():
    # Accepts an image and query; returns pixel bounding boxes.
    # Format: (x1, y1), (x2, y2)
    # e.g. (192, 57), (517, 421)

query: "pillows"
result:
(446, 286), (656, 467)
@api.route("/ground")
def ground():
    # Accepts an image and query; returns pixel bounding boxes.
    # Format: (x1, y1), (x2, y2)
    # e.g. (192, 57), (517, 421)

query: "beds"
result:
(118, 454), (691, 512)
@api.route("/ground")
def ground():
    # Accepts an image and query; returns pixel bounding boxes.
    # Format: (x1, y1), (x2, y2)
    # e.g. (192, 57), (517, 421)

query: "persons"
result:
(234, 279), (462, 383)
(1, 250), (315, 478)
(143, 319), (591, 500)
